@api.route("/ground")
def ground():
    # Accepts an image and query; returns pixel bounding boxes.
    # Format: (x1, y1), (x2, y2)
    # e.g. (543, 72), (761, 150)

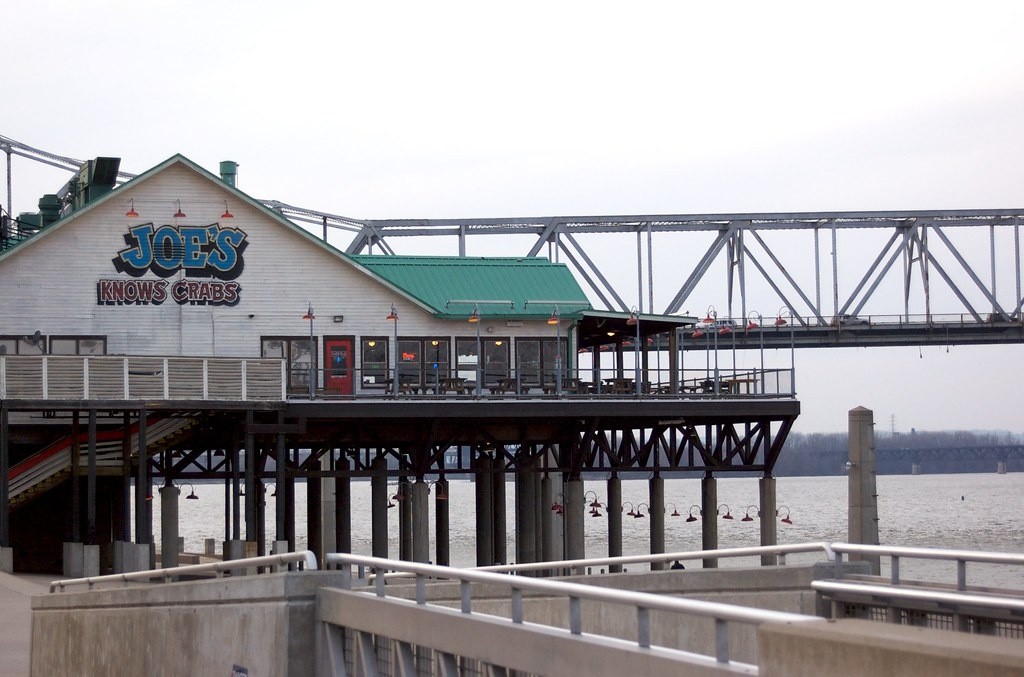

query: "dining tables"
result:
(552, 378), (580, 395)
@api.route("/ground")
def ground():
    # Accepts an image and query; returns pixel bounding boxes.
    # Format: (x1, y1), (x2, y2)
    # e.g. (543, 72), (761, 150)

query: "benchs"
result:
(398, 385), (430, 395)
(488, 386), (530, 395)
(431, 385), (475, 396)
(542, 386), (580, 394)
(653, 385), (702, 394)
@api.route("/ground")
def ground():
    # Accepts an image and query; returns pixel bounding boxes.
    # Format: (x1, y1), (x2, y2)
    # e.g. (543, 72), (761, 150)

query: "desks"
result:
(631, 381), (651, 394)
(581, 381), (602, 394)
(439, 377), (468, 395)
(699, 381), (729, 393)
(602, 378), (633, 394)
(496, 378), (526, 395)
(383, 379), (414, 400)
